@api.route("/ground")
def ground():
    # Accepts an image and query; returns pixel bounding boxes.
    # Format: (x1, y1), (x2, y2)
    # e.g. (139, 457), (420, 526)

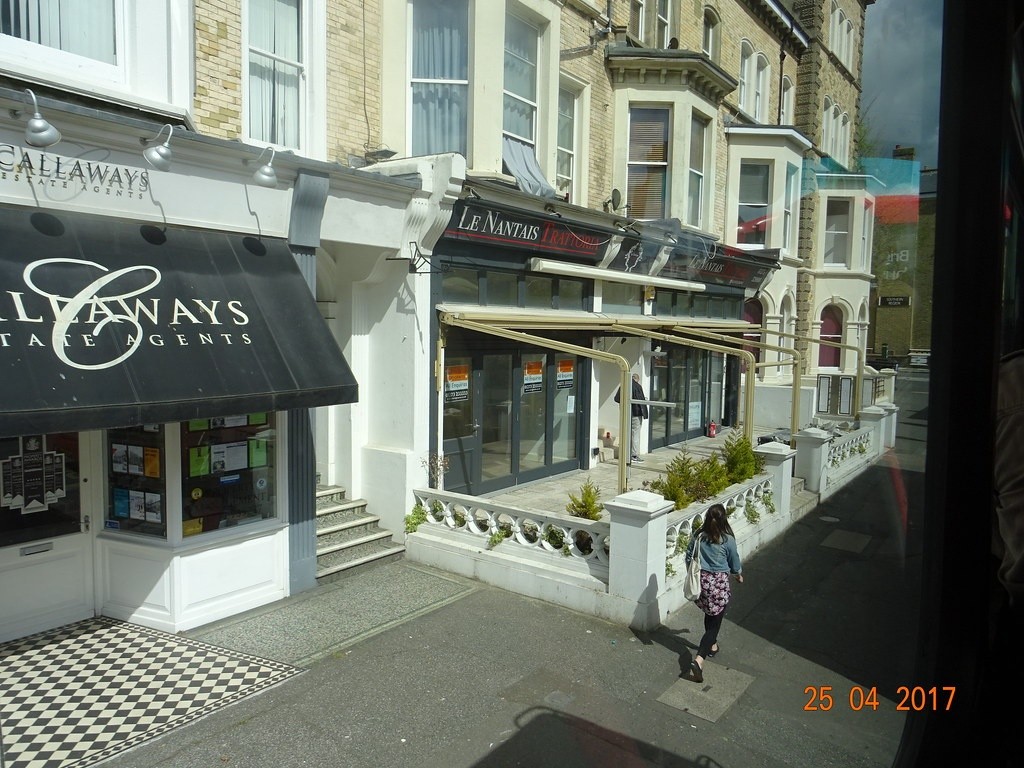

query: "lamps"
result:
(139, 123), (173, 171)
(9, 88), (63, 149)
(242, 146), (282, 189)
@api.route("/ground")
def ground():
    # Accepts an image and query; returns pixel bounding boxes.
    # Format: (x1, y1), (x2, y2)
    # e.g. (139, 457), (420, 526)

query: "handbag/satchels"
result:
(683, 532), (706, 601)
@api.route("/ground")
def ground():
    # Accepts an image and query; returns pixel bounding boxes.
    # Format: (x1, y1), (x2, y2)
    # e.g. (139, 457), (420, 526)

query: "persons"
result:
(614, 373), (648, 464)
(685, 504), (744, 683)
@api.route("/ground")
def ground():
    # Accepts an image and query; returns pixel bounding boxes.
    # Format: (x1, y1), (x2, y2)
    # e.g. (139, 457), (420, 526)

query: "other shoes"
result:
(690, 660), (704, 683)
(708, 645), (720, 657)
(631, 457), (645, 463)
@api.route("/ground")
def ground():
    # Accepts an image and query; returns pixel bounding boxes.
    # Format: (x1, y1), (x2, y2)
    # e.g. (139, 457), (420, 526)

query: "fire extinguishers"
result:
(709, 419), (716, 438)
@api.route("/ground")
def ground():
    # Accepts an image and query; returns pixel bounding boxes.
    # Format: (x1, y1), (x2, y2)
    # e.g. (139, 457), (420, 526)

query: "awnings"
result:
(0, 202), (359, 439)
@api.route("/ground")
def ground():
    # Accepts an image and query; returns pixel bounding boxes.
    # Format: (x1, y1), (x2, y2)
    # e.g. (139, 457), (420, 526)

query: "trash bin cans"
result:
(757, 430), (791, 445)
(866, 358), (899, 391)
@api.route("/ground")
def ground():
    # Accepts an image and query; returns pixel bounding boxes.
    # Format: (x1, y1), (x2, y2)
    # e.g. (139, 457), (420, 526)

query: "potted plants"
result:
(563, 475), (606, 551)
